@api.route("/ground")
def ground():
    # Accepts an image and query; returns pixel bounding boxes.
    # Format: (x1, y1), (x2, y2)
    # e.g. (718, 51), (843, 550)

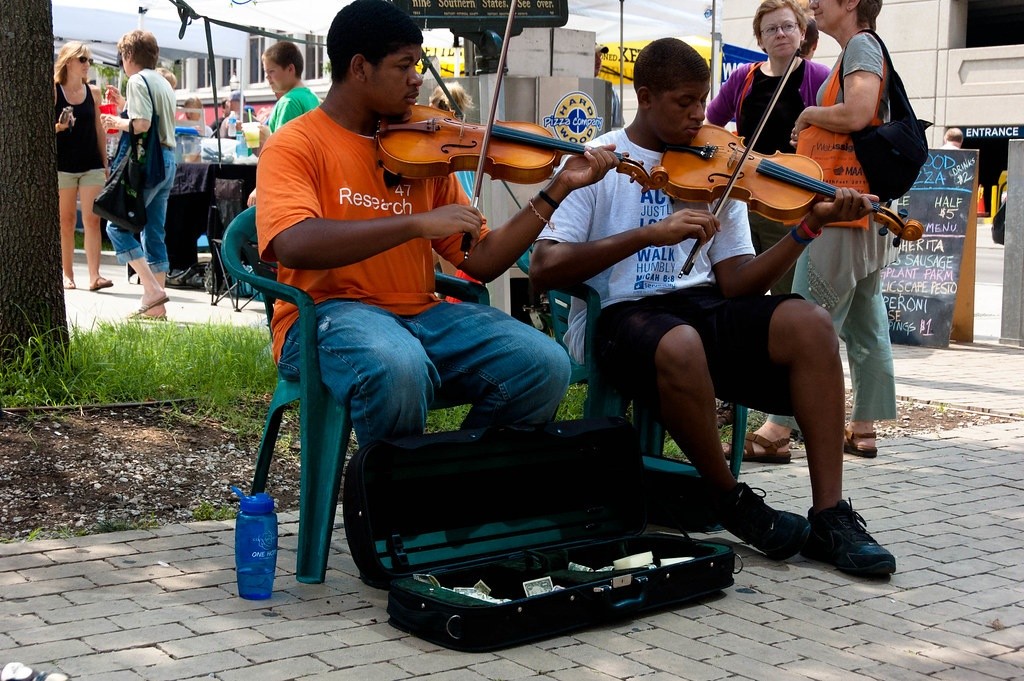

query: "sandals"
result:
(722, 430), (791, 464)
(843, 427), (878, 457)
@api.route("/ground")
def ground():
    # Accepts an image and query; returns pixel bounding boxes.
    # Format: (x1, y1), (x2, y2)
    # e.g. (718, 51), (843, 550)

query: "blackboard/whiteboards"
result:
(879, 148), (980, 348)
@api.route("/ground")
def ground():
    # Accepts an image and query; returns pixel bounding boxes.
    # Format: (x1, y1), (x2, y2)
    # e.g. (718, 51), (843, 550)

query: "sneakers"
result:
(719, 482), (812, 562)
(801, 495), (897, 578)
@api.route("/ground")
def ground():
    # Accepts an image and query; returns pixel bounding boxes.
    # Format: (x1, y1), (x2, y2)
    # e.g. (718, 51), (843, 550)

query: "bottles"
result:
(232, 487), (278, 599)
(227, 111), (237, 137)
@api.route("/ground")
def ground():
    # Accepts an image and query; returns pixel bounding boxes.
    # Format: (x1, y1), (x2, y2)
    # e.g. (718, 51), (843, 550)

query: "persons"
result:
(595, 42), (622, 133)
(705, 0), (896, 463)
(528, 37), (895, 577)
(429, 80), (477, 302)
(941, 127), (965, 150)
(1000, 189), (1008, 204)
(154, 41), (321, 208)
(99, 30), (177, 321)
(254, 1), (572, 448)
(53, 41), (114, 291)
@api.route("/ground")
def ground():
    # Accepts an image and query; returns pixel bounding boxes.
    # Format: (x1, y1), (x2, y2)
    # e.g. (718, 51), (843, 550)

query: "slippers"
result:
(90, 276), (114, 292)
(126, 293), (170, 321)
(63, 279), (76, 289)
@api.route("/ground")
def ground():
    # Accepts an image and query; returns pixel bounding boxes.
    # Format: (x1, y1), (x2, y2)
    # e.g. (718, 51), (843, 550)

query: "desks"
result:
(125, 161), (256, 299)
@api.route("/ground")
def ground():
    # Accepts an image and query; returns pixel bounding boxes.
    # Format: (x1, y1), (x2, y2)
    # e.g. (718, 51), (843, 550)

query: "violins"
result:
(376, 103), (668, 190)
(662, 123), (924, 247)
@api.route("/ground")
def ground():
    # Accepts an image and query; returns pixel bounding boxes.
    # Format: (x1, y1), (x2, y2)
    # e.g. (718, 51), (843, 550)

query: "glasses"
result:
(760, 22), (797, 36)
(79, 56), (93, 66)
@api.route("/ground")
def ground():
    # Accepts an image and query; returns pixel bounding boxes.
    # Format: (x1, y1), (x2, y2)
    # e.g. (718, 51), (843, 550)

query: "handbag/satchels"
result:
(840, 29), (929, 204)
(793, 119), (883, 232)
(110, 110), (166, 189)
(92, 119), (148, 234)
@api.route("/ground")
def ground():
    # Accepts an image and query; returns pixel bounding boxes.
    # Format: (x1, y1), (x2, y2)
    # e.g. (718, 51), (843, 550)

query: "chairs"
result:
(202, 177), (265, 313)
(516, 224), (749, 500)
(218, 206), (493, 587)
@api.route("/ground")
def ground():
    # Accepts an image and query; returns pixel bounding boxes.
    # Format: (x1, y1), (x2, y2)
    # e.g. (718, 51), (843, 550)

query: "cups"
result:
(241, 123), (260, 149)
(98, 104), (119, 133)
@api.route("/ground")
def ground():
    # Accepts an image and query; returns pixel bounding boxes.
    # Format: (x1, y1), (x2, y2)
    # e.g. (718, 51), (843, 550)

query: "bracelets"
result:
(539, 191), (560, 210)
(58, 124), (64, 131)
(800, 220), (821, 238)
(529, 198), (555, 232)
(791, 225), (814, 245)
